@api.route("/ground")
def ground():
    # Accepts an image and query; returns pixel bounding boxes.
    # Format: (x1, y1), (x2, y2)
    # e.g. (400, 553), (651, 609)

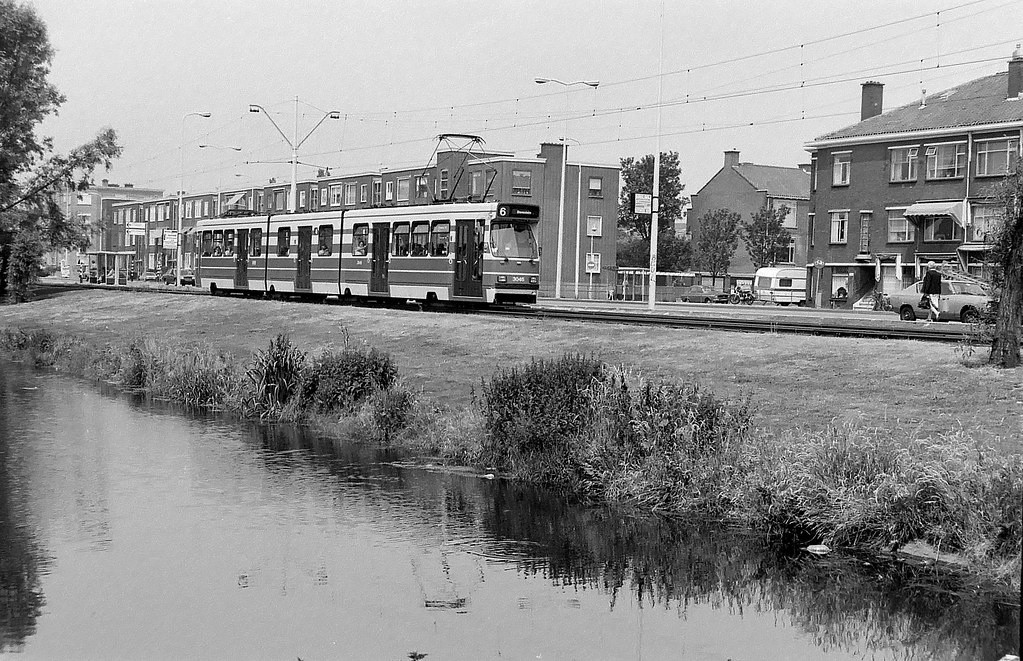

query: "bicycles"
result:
(868, 290), (890, 311)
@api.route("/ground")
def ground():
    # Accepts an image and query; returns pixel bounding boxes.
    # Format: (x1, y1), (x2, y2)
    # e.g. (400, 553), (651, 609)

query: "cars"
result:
(140, 272), (157, 280)
(681, 286), (730, 304)
(162, 268), (195, 287)
(890, 278), (999, 323)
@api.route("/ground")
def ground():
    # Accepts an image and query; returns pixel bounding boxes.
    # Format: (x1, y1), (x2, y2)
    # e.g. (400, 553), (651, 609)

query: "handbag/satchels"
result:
(917, 294), (931, 309)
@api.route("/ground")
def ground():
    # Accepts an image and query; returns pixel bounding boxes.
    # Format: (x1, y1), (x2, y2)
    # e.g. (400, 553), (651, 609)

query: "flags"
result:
(177, 227), (192, 236)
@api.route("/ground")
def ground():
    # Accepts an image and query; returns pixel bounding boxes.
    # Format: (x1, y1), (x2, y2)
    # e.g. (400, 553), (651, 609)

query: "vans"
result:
(753, 267), (807, 308)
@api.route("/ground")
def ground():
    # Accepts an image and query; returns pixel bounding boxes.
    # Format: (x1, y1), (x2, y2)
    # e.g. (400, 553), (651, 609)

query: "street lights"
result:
(176, 112), (210, 286)
(250, 103), (341, 213)
(199, 143), (243, 216)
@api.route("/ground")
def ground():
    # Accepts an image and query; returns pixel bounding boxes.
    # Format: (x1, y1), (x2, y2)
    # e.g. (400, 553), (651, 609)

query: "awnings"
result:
(226, 193), (248, 207)
(902, 202), (966, 229)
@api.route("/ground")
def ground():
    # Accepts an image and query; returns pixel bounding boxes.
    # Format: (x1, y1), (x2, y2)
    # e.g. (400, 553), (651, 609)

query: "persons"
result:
(211, 245), (233, 257)
(399, 242), (446, 256)
(354, 241), (367, 255)
(318, 244), (330, 255)
(920, 261), (941, 321)
(91, 260), (97, 269)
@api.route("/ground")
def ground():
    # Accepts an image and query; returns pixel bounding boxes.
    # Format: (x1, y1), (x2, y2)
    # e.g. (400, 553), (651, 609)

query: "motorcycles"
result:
(731, 284), (756, 306)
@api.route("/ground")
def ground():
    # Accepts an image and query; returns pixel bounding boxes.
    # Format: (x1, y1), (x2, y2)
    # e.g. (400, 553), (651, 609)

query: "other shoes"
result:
(936, 311), (941, 320)
(923, 319), (932, 322)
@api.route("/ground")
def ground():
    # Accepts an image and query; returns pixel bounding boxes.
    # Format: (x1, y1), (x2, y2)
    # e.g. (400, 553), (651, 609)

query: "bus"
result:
(193, 203), (542, 304)
(617, 269), (698, 303)
